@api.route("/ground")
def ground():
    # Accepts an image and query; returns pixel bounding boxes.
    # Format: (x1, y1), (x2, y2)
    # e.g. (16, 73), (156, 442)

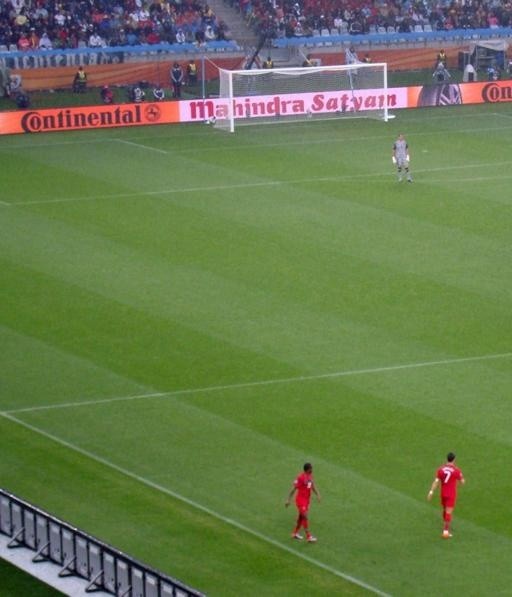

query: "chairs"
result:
(0, 0), (512, 53)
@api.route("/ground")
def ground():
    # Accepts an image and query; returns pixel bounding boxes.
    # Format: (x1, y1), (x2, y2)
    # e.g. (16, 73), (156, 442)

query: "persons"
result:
(1, 0), (512, 106)
(425, 450), (465, 538)
(389, 133), (413, 181)
(283, 461), (320, 544)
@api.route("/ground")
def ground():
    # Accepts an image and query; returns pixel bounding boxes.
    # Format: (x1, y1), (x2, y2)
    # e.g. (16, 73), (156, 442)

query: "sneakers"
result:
(291, 532), (304, 540)
(306, 536), (318, 542)
(442, 534), (453, 538)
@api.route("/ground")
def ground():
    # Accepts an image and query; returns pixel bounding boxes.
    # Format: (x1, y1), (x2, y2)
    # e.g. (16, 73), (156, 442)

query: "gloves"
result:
(392, 156), (397, 164)
(406, 154), (409, 161)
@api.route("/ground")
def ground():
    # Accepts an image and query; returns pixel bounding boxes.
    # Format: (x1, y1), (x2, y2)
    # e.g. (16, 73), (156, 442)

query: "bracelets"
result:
(427, 489), (432, 494)
